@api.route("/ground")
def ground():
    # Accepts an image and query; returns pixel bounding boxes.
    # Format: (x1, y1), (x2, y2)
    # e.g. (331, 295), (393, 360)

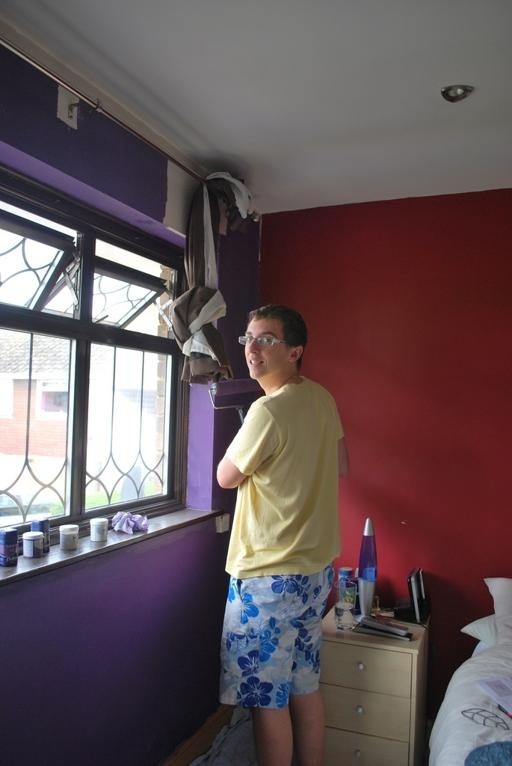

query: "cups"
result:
(334, 581), (357, 629)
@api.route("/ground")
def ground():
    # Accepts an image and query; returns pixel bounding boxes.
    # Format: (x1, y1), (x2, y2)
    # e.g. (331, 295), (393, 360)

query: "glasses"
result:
(237, 334), (286, 348)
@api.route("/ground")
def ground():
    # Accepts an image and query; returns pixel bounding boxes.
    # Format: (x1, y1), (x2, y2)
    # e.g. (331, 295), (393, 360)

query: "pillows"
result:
(460, 614), (498, 647)
(483, 577), (512, 645)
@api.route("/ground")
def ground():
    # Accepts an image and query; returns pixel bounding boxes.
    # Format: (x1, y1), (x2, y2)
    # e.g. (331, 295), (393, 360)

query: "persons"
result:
(216, 301), (350, 765)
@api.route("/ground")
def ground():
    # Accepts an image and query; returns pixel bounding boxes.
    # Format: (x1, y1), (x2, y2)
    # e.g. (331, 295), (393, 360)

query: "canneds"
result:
(339, 567), (352, 598)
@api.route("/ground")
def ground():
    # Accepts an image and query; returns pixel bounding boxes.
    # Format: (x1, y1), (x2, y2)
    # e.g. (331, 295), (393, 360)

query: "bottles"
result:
(337, 567), (353, 602)
(23, 532), (44, 558)
(90, 518), (108, 542)
(59, 524), (79, 551)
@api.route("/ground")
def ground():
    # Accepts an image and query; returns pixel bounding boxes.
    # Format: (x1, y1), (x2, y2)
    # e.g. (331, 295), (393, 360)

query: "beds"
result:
(429, 638), (511, 766)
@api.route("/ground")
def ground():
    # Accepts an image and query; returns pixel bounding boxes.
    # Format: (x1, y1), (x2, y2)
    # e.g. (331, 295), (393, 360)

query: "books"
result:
(477, 673), (512, 718)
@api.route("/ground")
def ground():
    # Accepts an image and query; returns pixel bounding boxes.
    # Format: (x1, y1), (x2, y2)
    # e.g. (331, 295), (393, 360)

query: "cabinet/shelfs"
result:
(319, 601), (431, 766)
